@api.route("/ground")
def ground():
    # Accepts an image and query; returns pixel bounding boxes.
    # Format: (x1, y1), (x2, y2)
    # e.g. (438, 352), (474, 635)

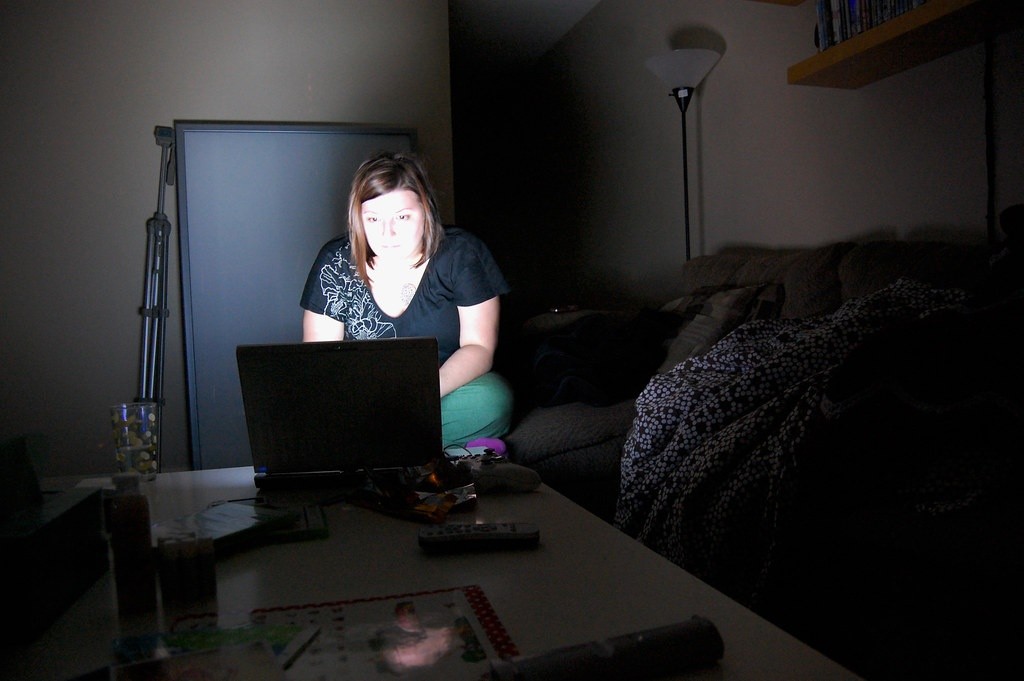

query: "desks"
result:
(1, 455), (866, 681)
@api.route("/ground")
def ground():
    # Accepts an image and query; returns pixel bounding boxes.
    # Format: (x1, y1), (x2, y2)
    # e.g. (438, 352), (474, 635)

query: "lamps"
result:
(643, 46), (722, 266)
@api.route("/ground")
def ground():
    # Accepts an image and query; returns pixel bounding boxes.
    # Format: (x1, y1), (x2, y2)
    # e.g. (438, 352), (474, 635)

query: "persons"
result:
(300, 152), (514, 451)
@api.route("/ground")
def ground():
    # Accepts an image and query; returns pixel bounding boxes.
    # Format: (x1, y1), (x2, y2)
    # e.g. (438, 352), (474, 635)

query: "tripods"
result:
(134, 125), (176, 472)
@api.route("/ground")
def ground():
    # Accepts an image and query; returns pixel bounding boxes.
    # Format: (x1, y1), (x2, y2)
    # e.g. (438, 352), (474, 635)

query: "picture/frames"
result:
(173, 116), (429, 476)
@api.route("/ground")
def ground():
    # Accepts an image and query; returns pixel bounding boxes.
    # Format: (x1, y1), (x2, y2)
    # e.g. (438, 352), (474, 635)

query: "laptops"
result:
(235, 336), (448, 488)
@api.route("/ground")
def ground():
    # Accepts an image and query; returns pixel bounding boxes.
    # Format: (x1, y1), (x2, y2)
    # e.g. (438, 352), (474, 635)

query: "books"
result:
(817, 0), (927, 52)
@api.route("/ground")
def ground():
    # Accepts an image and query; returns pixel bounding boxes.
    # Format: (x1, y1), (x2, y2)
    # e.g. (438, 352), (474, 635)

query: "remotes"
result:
(418, 523), (540, 550)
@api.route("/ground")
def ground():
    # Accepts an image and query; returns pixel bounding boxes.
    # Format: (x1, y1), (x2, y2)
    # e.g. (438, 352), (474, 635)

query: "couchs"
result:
(501, 231), (1023, 681)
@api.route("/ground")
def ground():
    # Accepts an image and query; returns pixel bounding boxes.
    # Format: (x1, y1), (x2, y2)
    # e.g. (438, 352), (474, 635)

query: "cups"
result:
(110, 402), (157, 481)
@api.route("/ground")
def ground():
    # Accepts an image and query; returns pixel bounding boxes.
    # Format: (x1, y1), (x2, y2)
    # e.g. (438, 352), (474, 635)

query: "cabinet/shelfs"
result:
(782, 0), (1024, 92)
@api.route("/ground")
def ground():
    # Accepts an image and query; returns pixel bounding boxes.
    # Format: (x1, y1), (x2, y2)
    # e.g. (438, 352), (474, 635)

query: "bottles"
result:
(96, 473), (217, 615)
